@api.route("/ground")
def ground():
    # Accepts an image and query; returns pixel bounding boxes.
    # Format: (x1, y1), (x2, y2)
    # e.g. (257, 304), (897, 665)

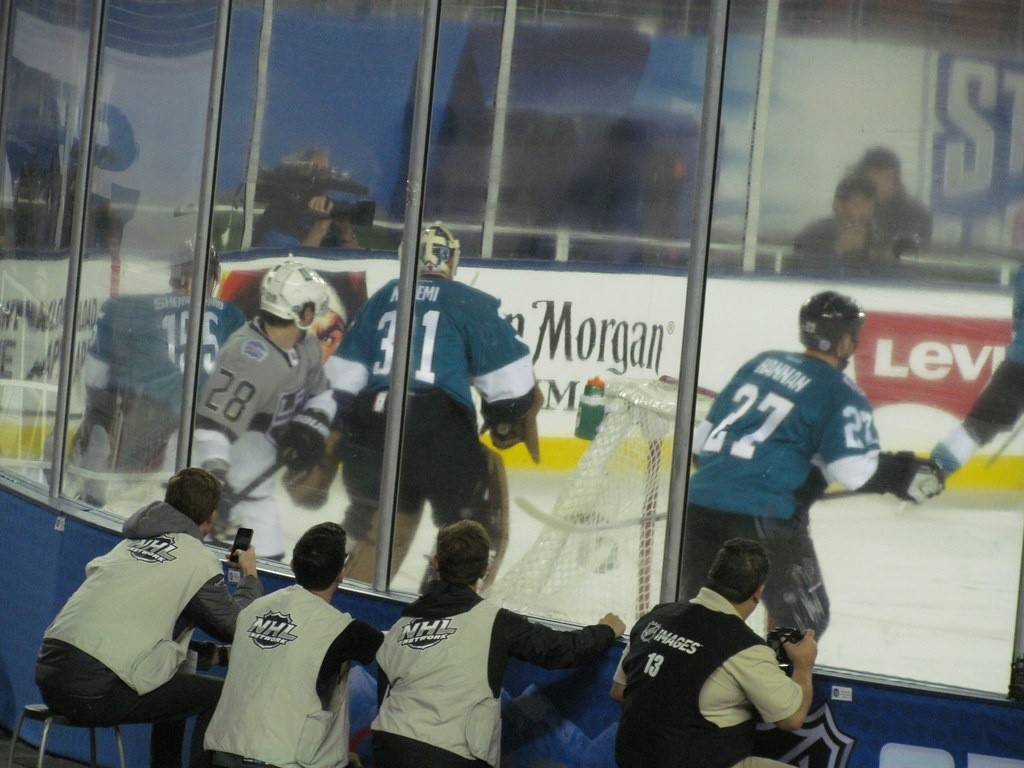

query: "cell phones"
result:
(230, 528), (254, 563)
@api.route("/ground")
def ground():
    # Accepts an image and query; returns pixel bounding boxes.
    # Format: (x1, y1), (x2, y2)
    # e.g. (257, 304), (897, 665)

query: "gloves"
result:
(879, 450), (946, 504)
(206, 469), (233, 516)
(276, 410), (331, 466)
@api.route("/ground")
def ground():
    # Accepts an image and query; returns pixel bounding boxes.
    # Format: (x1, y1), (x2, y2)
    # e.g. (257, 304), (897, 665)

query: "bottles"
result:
(575, 375), (605, 440)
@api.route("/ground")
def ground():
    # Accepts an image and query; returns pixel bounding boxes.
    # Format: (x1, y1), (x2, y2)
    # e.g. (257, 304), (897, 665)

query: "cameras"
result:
(767, 626), (805, 665)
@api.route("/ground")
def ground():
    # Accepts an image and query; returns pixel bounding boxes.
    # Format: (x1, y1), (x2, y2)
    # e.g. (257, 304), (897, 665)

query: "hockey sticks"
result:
(987, 418), (1023, 470)
(514, 489), (861, 534)
(496, 422), (510, 440)
(234, 459), (288, 503)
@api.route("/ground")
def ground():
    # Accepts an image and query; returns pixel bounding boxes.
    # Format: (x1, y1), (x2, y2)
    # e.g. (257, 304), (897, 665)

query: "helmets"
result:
(170, 238), (221, 290)
(798, 290), (866, 353)
(258, 259), (334, 330)
(393, 220), (459, 277)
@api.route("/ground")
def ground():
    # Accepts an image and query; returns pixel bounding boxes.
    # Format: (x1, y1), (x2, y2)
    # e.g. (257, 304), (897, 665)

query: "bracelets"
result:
(341, 234), (357, 242)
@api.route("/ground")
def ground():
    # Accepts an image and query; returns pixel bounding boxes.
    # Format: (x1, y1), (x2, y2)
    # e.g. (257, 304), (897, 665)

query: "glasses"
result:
(342, 554), (350, 565)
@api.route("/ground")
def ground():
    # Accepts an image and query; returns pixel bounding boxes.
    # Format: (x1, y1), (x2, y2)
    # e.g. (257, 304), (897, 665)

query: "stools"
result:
(9, 703), (127, 768)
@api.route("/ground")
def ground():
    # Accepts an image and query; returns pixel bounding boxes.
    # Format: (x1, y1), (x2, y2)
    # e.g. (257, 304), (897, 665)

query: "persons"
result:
(254, 146), (361, 250)
(36, 467), (263, 768)
(326, 220), (535, 588)
(366, 520), (626, 768)
(525, 113), (653, 267)
(791, 143), (932, 284)
(927, 259), (1024, 698)
(236, 270), (352, 365)
(675, 291), (944, 665)
(65, 236), (244, 508)
(610, 538), (817, 768)
(164, 261), (331, 562)
(200, 522), (390, 768)
